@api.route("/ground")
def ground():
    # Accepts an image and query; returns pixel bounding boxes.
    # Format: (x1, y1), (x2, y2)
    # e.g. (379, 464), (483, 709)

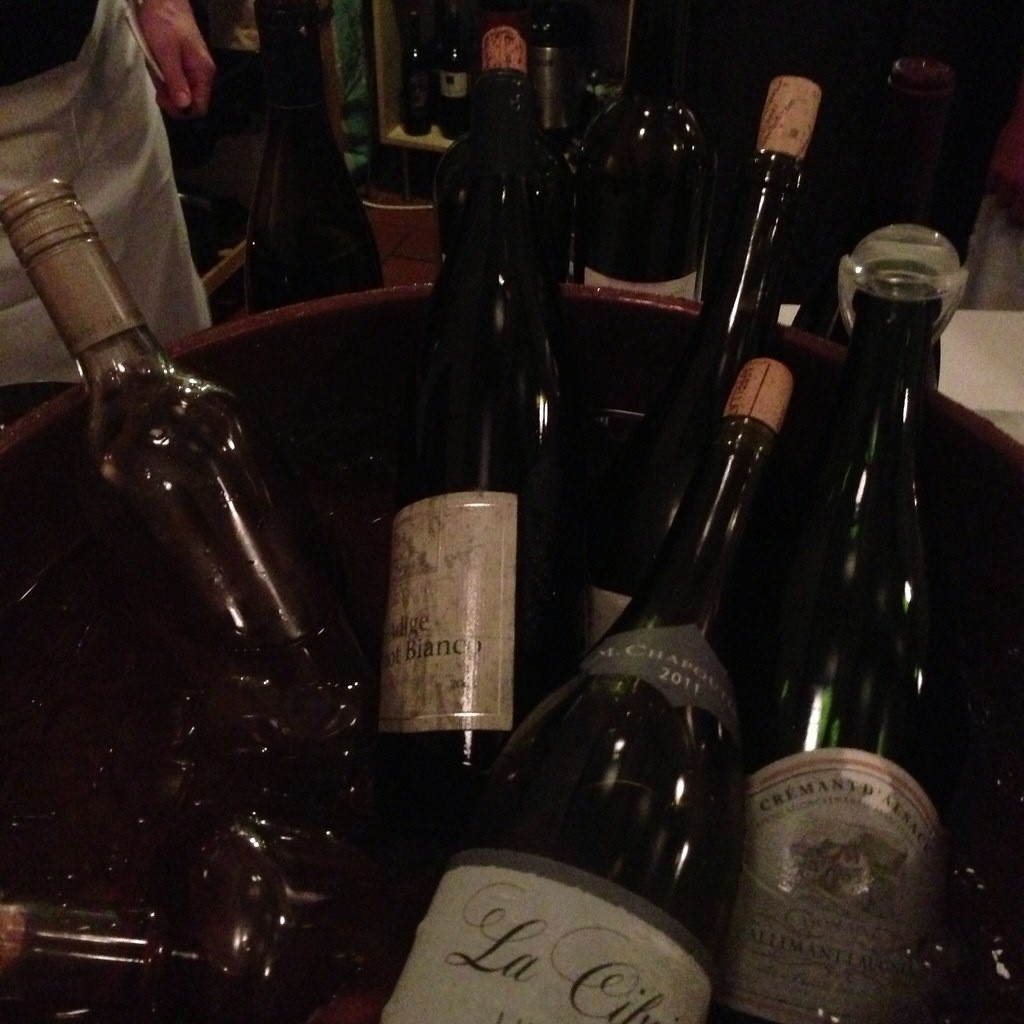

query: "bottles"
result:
(0, 0), (1024, 1024)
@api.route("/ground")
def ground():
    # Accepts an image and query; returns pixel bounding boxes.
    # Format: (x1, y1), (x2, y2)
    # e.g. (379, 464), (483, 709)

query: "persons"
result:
(958, 75), (1023, 311)
(0, 0), (216, 421)
